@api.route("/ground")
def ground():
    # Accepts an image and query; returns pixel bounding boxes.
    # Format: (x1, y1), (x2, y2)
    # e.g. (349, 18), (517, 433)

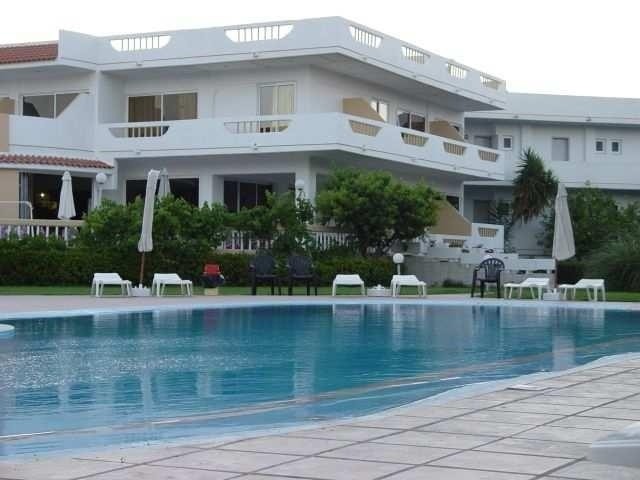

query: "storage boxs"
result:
(204, 286), (218, 295)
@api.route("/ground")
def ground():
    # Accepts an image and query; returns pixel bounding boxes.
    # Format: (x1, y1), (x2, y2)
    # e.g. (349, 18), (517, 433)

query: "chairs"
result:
(248, 253), (427, 298)
(471, 258), (606, 301)
(91, 272), (192, 298)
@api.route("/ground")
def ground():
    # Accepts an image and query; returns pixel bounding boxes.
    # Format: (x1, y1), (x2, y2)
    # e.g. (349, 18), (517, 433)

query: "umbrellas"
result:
(552, 180), (576, 289)
(136, 169), (159, 285)
(58, 170), (77, 242)
(157, 166), (172, 202)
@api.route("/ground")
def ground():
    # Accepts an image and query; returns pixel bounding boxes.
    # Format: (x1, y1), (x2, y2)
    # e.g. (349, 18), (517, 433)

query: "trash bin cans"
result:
(200, 264), (225, 296)
